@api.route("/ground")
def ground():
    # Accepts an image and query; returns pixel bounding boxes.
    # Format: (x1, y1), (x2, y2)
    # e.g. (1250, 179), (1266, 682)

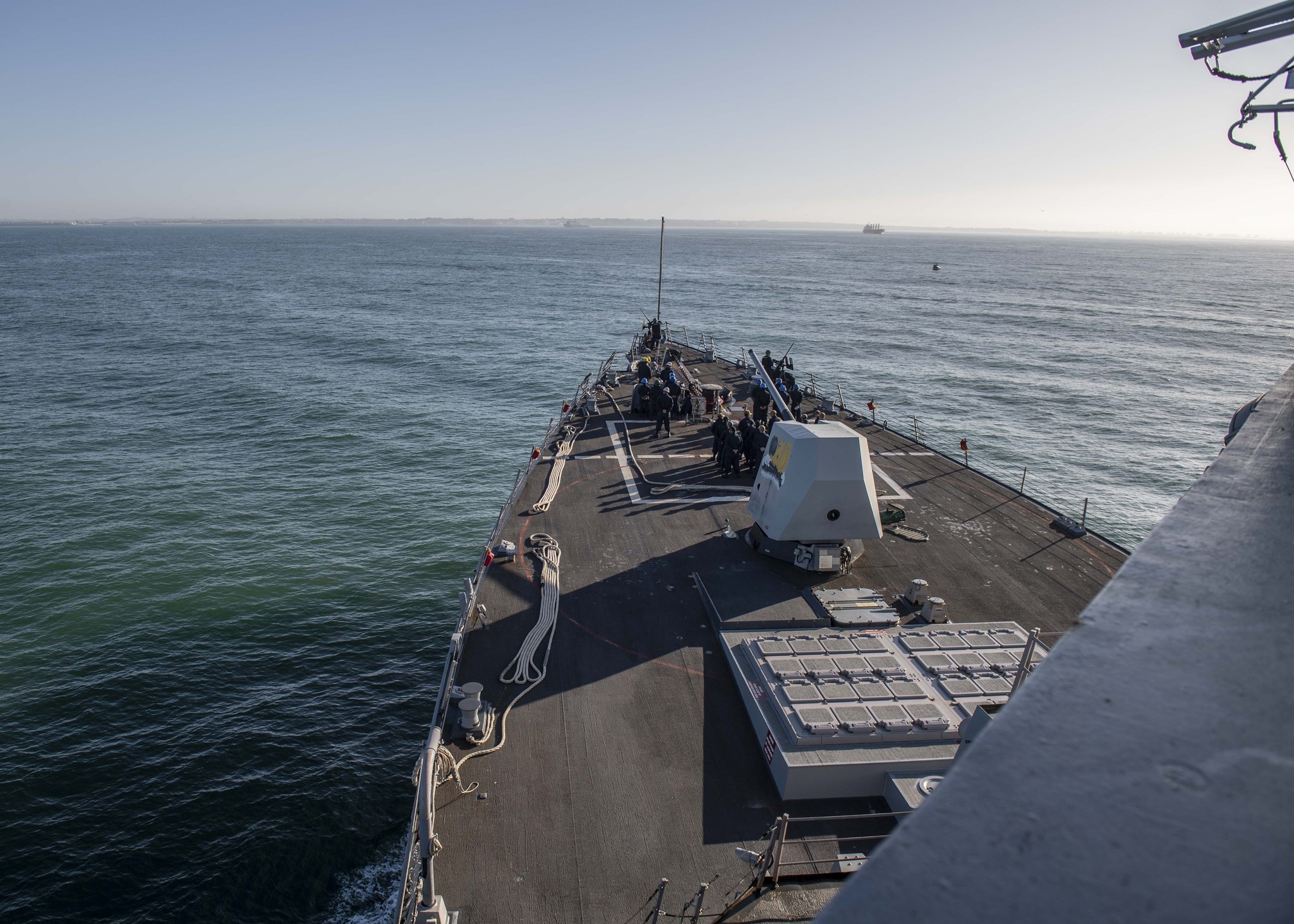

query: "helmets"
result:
(669, 376), (676, 382)
(643, 357), (649, 362)
(669, 373), (675, 377)
(653, 318), (657, 322)
(765, 350), (771, 355)
(665, 362), (673, 367)
(641, 378), (648, 383)
(646, 356), (651, 360)
(654, 380), (661, 386)
(756, 377), (800, 391)
(662, 387), (670, 393)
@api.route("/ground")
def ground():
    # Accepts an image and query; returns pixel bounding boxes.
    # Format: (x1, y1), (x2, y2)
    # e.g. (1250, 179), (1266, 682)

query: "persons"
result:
(666, 376), (683, 420)
(653, 387), (674, 438)
(648, 380), (663, 420)
(711, 377), (826, 479)
(638, 357), (652, 384)
(637, 378), (651, 414)
(762, 350), (774, 378)
(647, 318), (663, 350)
(647, 356), (651, 363)
(663, 373), (675, 390)
(660, 362), (675, 384)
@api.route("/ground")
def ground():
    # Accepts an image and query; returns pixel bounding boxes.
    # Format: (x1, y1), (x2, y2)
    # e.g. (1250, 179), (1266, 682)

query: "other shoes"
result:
(653, 431), (658, 438)
(710, 453), (758, 480)
(667, 431), (671, 438)
(649, 347), (653, 350)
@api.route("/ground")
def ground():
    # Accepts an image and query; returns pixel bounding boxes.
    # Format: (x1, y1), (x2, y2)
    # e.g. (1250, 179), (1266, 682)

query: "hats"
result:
(717, 409), (825, 431)
